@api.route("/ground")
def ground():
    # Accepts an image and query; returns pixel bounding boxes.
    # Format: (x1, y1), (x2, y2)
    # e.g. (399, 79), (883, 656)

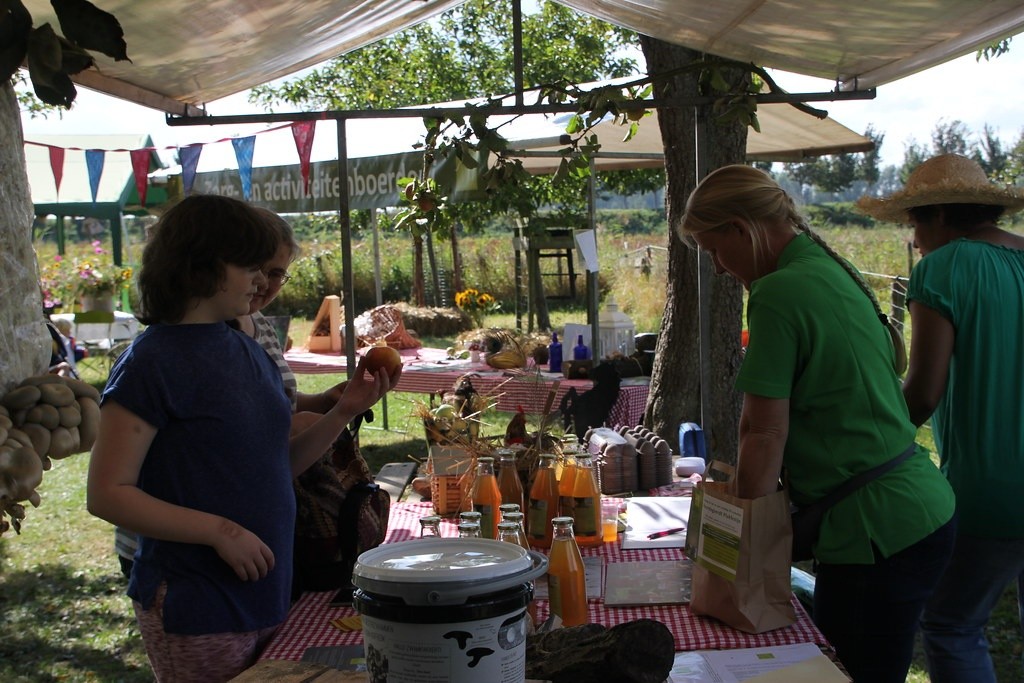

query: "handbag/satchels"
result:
(791, 442), (920, 563)
(683, 460), (798, 633)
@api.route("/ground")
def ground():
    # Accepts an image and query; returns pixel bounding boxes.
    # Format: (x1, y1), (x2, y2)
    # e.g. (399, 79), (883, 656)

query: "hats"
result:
(852, 154), (1024, 224)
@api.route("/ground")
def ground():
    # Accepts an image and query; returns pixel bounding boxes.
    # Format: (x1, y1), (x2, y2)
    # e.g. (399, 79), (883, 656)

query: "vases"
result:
(80, 296), (113, 314)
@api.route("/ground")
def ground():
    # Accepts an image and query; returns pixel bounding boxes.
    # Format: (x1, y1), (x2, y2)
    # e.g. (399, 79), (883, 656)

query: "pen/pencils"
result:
(647, 528), (684, 540)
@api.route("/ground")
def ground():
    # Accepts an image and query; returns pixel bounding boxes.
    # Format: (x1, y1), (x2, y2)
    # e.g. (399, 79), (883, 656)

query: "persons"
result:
(55, 319), (76, 349)
(677, 164), (957, 679)
(115, 208), (351, 580)
(852, 154), (1024, 683)
(87, 195), (403, 683)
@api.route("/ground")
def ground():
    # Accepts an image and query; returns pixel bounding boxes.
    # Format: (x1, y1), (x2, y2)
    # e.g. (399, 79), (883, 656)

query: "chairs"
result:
(72, 313), (116, 380)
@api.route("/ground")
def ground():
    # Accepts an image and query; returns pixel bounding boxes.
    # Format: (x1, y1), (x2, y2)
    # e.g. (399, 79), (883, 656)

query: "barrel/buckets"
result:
(349, 537), (550, 683)
(265, 316), (290, 353)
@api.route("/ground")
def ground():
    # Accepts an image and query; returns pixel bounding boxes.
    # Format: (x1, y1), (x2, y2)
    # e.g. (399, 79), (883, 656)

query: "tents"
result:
(151, 69), (874, 367)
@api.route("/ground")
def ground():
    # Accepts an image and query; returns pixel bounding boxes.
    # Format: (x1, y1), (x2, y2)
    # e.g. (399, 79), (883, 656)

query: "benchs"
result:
(375, 461), (430, 504)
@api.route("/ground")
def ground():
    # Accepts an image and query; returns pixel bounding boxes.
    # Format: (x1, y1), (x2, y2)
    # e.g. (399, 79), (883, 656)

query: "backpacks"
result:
(292, 427), (390, 591)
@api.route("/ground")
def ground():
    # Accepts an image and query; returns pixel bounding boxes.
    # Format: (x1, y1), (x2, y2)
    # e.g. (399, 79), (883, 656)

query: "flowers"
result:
(40, 239), (132, 314)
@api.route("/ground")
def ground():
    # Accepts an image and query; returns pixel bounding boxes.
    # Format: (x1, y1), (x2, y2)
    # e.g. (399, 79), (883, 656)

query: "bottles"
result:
(526, 454), (559, 550)
(572, 453), (604, 546)
(572, 334), (591, 360)
(548, 517), (588, 627)
(471, 455), (502, 540)
(549, 332), (563, 373)
(419, 504), (536, 629)
(495, 450), (526, 531)
(558, 450), (578, 523)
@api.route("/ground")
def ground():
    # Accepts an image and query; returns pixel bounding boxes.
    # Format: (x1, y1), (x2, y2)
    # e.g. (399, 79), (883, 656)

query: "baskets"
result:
(426, 453), (473, 518)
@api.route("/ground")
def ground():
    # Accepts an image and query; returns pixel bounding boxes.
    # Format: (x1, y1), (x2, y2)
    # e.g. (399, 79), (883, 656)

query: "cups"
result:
(598, 496), (620, 542)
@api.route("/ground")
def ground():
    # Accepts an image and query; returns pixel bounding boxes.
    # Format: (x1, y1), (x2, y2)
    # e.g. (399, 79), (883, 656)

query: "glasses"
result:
(260, 268), (291, 287)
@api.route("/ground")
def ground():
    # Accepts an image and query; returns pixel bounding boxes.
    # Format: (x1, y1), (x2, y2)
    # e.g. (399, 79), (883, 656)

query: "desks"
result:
(50, 310), (140, 339)
(235, 498), (855, 683)
(286, 345), (651, 434)
